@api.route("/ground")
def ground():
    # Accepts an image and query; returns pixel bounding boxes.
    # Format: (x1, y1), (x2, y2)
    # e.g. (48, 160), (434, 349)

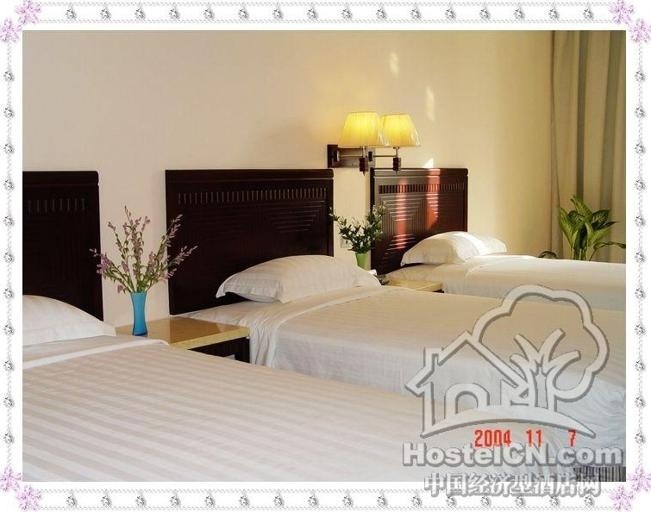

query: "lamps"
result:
(326, 112), (422, 176)
